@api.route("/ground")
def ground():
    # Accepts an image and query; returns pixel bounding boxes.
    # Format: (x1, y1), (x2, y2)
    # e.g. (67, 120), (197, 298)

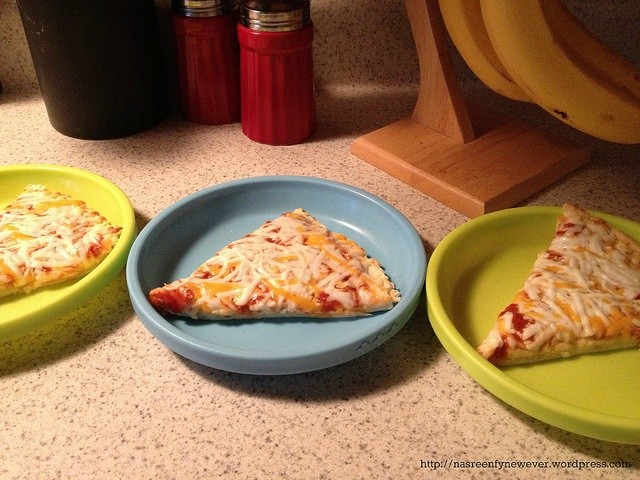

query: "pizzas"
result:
(476, 200), (640, 370)
(147, 206), (404, 319)
(1, 179), (122, 302)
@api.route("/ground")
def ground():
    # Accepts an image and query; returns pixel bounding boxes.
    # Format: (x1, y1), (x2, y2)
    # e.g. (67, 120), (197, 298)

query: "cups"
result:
(237, 0), (316, 144)
(174, 0), (239, 125)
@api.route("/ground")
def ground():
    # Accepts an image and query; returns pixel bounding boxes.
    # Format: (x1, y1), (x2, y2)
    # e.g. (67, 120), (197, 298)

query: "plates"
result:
(425, 205), (640, 447)
(0, 163), (137, 339)
(126, 175), (428, 377)
